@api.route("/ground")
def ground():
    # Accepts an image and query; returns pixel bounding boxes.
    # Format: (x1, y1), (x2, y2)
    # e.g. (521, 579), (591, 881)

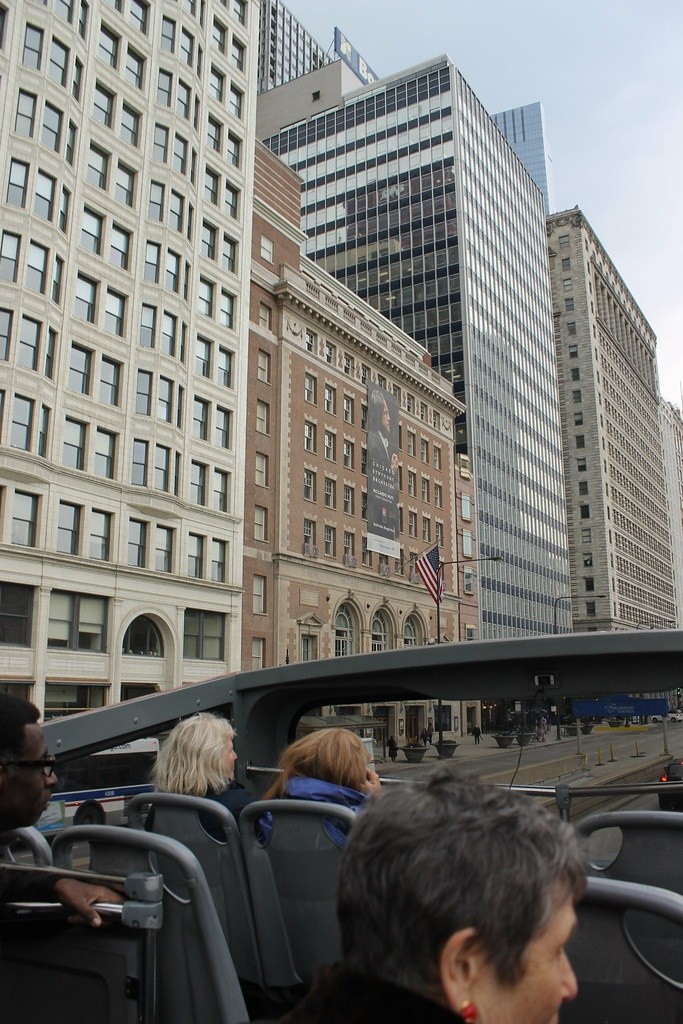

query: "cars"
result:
(651, 709), (683, 722)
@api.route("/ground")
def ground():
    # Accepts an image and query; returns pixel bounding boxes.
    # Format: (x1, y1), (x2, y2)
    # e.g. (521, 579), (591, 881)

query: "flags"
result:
(415, 545), (443, 605)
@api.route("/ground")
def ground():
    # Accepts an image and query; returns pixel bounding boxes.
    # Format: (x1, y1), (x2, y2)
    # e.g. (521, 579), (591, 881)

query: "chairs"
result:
(0, 793), (683, 1024)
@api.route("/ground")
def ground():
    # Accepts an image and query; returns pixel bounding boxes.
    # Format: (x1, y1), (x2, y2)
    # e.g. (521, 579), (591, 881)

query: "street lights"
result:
(554, 594), (607, 739)
(637, 620), (675, 725)
(437, 557), (503, 760)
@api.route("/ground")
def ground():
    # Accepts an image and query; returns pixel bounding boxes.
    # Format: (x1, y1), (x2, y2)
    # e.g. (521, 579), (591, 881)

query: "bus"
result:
(33, 737), (159, 836)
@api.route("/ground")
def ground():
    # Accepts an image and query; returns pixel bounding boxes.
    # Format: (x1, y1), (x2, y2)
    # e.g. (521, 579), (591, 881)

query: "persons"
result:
(420, 722), (433, 746)
(0, 691), (128, 1024)
(260, 724), (382, 848)
(144, 711), (261, 847)
(470, 722), (481, 746)
(386, 736), (399, 762)
(274, 768), (592, 1024)
(540, 714), (552, 733)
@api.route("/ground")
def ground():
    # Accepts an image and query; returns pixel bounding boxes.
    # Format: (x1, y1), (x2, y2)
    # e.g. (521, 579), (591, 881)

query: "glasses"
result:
(0, 754), (56, 777)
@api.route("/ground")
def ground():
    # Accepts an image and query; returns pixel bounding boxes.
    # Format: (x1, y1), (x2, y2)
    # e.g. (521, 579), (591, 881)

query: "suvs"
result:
(658, 757), (683, 810)
